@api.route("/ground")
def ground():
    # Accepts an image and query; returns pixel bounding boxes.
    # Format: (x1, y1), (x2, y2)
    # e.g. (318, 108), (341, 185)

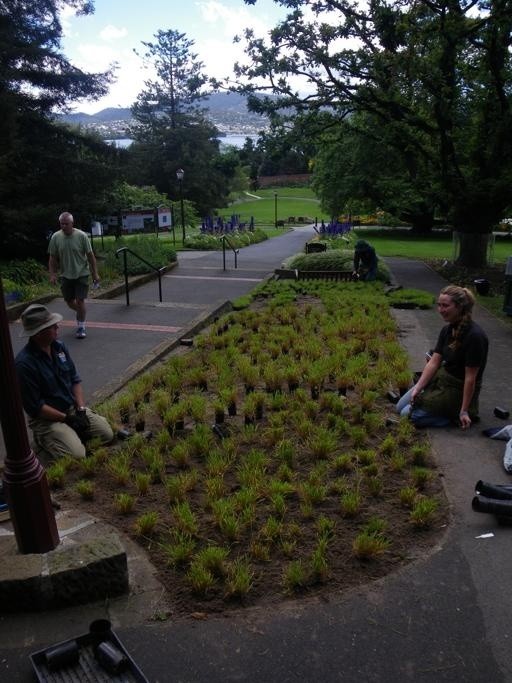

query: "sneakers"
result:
(74, 327), (87, 338)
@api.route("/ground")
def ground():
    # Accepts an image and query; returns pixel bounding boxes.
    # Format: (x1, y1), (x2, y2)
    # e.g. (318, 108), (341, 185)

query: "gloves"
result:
(76, 410), (91, 428)
(62, 414), (85, 434)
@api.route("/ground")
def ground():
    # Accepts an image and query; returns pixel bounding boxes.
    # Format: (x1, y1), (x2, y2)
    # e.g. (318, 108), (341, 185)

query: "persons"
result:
(395, 283), (489, 429)
(350, 239), (378, 281)
(13, 302), (115, 473)
(48, 210), (101, 338)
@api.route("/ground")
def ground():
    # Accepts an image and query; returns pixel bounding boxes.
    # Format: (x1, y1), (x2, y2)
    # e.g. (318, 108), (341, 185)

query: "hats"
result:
(354, 239), (370, 250)
(18, 303), (64, 338)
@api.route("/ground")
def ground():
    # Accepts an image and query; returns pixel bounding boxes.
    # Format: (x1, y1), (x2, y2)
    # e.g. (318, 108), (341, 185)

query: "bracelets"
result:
(459, 410), (468, 416)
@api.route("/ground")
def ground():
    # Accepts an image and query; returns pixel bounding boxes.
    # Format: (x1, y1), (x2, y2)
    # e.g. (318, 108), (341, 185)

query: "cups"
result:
(89, 618), (127, 672)
(45, 640), (81, 668)
(179, 338), (193, 345)
(493, 407), (510, 420)
(472, 477), (512, 513)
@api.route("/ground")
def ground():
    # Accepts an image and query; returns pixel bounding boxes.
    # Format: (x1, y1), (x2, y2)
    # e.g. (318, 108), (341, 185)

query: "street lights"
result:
(273, 189), (279, 228)
(175, 167), (186, 246)
